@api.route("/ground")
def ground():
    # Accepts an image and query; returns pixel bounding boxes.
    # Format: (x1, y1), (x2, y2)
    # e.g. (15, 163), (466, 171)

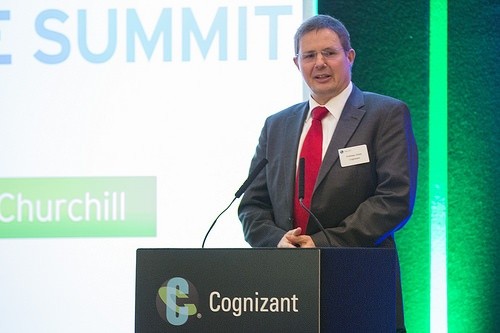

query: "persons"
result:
(239, 14), (419, 333)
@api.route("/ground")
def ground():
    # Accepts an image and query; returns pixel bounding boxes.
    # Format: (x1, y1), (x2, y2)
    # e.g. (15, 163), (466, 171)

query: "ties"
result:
(293, 106), (329, 247)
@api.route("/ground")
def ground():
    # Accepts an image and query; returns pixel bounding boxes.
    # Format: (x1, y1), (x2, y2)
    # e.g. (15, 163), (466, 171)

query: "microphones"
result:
(298, 157), (333, 247)
(202, 158), (269, 248)
